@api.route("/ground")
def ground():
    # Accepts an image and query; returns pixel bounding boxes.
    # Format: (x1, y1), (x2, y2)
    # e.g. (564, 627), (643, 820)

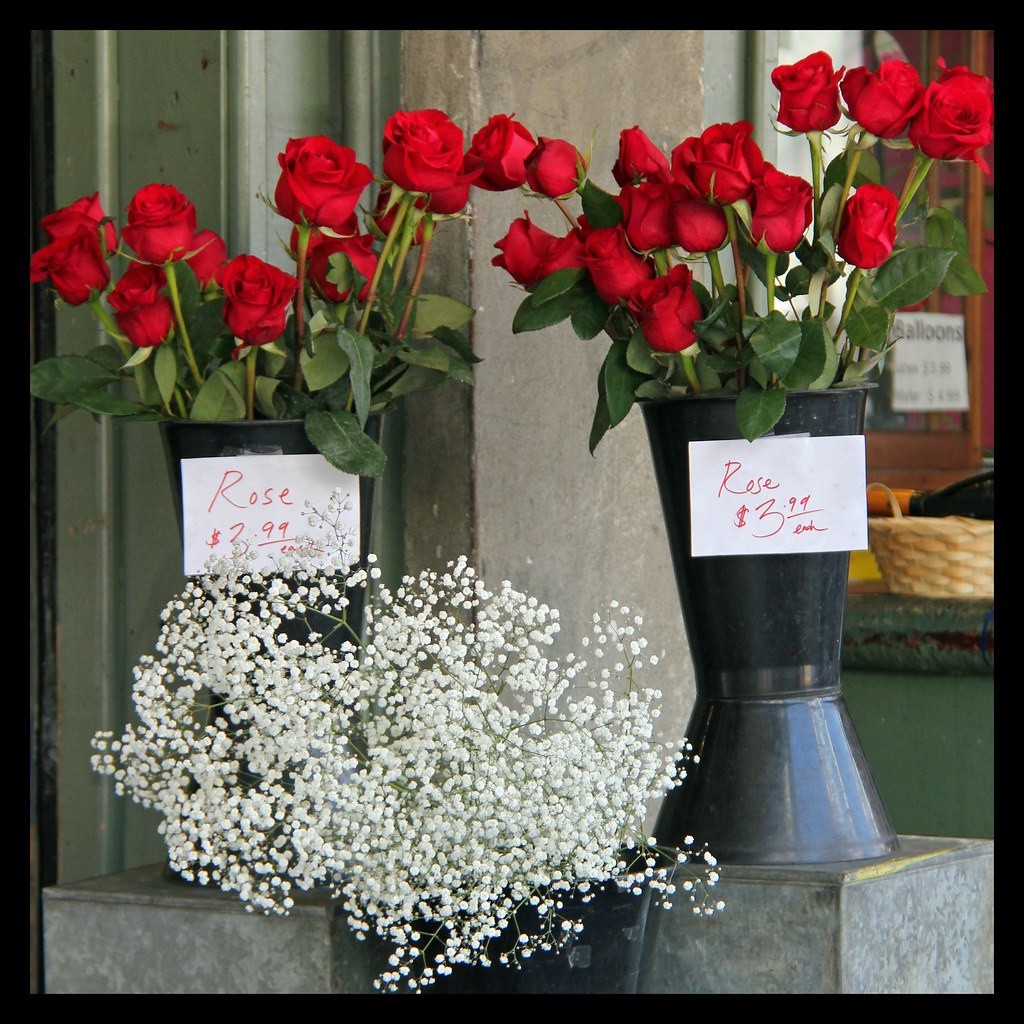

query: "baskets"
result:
(866, 481), (993, 598)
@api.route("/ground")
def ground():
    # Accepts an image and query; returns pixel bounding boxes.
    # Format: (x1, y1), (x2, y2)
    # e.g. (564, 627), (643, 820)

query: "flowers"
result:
(465, 50), (994, 458)
(89, 489), (726, 993)
(31, 52), (484, 480)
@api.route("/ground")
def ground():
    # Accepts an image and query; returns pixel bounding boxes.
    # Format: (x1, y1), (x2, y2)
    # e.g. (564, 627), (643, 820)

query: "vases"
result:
(154, 419), (382, 886)
(635, 385), (905, 863)
(412, 847), (687, 993)
